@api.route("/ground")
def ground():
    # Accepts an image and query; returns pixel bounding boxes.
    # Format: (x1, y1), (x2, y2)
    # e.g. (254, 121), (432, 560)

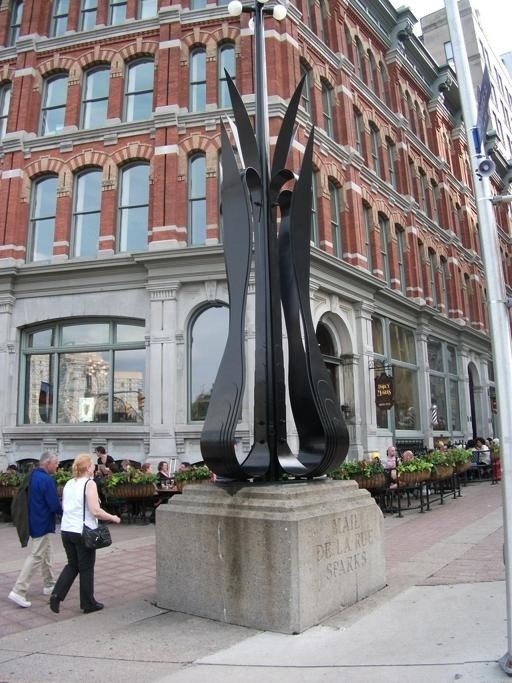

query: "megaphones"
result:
(478, 158), (496, 176)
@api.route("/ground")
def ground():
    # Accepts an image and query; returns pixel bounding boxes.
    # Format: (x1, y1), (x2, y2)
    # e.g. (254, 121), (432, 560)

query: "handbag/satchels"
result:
(83, 523), (112, 550)
(8, 493), (30, 547)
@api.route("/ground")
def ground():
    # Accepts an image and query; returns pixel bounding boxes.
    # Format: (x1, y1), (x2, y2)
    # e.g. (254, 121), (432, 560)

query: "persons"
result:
(8, 451), (62, 607)
(386, 437), (501, 492)
(50, 454), (121, 614)
(6, 446), (216, 496)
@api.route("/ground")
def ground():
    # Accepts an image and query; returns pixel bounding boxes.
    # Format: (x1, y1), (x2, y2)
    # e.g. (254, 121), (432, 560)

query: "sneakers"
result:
(50, 593), (59, 613)
(84, 603), (104, 613)
(43, 586), (55, 595)
(7, 591), (32, 608)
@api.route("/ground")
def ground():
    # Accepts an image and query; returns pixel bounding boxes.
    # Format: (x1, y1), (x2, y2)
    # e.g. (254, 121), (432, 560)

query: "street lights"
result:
(227, 0), (286, 481)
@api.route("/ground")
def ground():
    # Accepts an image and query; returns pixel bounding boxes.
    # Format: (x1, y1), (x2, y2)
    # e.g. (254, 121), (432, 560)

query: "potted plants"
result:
(332, 438), (502, 490)
(0, 465), (214, 498)
